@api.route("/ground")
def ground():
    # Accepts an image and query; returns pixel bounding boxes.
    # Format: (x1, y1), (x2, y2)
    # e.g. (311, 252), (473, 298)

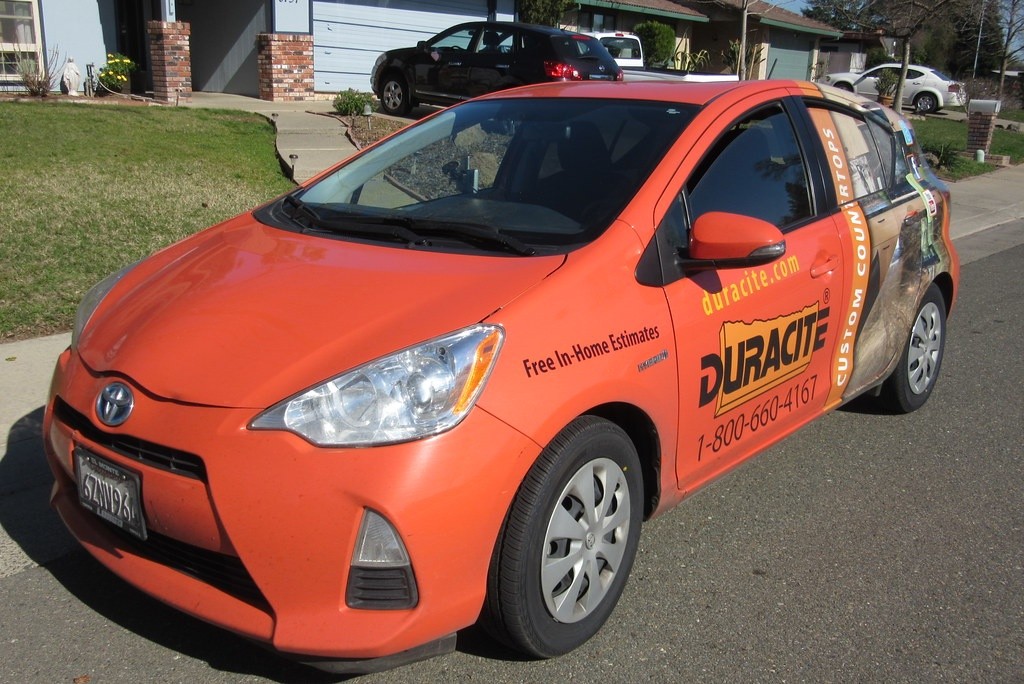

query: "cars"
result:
(38, 76), (957, 679)
(368, 21), (624, 115)
(818, 62), (967, 115)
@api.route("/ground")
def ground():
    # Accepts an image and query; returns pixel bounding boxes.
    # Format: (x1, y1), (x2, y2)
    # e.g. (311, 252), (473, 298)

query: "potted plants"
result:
(633, 20), (676, 70)
(873, 67), (899, 108)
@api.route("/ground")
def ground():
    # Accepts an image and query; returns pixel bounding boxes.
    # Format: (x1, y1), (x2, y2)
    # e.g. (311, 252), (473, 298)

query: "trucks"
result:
(570, 30), (742, 84)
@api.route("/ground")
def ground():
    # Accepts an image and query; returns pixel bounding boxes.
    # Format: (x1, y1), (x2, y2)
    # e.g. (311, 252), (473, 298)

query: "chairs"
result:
(476, 29), (502, 69)
(543, 115), (639, 229)
